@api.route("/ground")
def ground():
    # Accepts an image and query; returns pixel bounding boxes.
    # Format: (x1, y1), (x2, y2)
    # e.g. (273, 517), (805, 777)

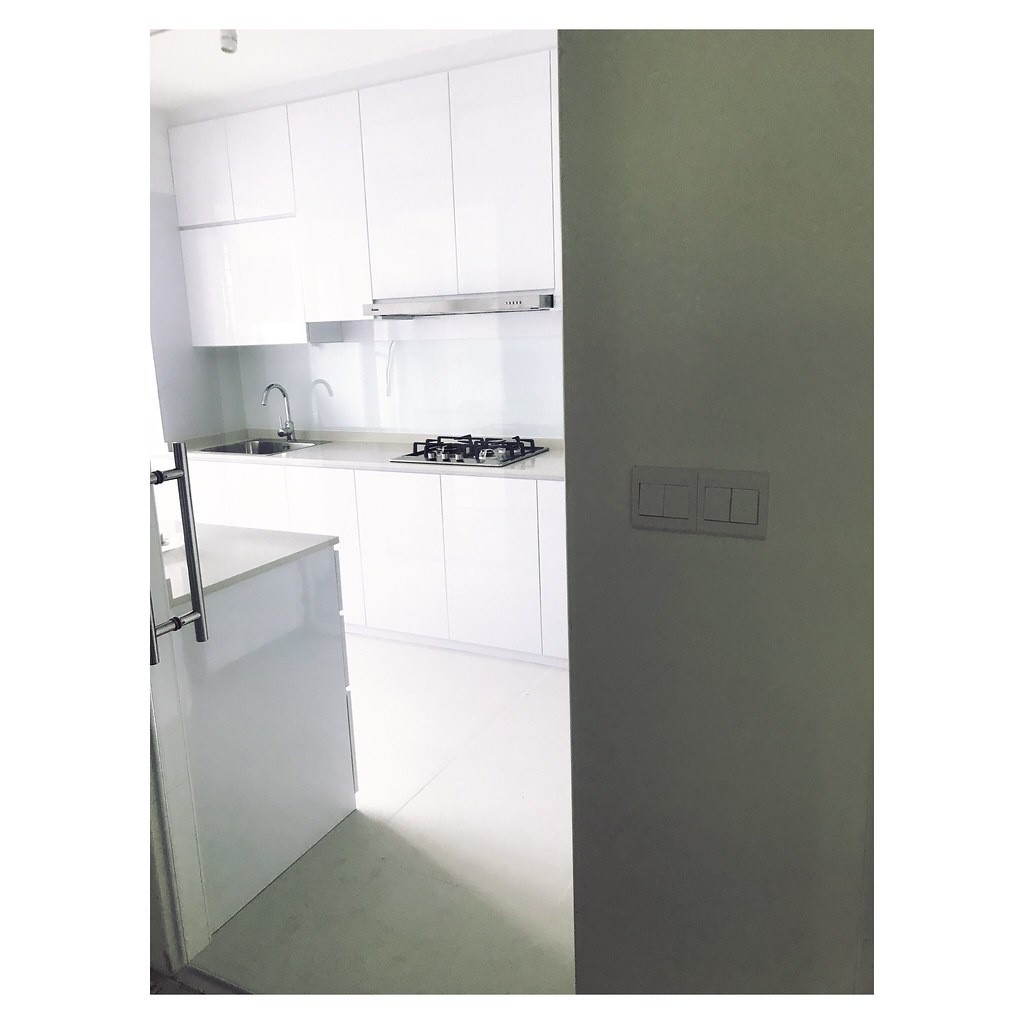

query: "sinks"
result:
(200, 438), (334, 457)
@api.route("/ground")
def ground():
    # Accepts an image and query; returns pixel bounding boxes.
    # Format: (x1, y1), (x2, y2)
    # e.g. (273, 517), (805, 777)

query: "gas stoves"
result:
(390, 434), (549, 467)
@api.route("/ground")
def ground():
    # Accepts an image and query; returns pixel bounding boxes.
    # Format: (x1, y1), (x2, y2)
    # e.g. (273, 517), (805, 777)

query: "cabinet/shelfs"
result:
(170, 547), (359, 935)
(166, 454), (569, 670)
(170, 45), (561, 346)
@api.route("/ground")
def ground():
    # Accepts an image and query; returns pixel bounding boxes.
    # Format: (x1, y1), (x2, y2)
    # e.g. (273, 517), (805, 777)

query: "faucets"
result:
(311, 379), (334, 426)
(261, 383), (296, 441)
